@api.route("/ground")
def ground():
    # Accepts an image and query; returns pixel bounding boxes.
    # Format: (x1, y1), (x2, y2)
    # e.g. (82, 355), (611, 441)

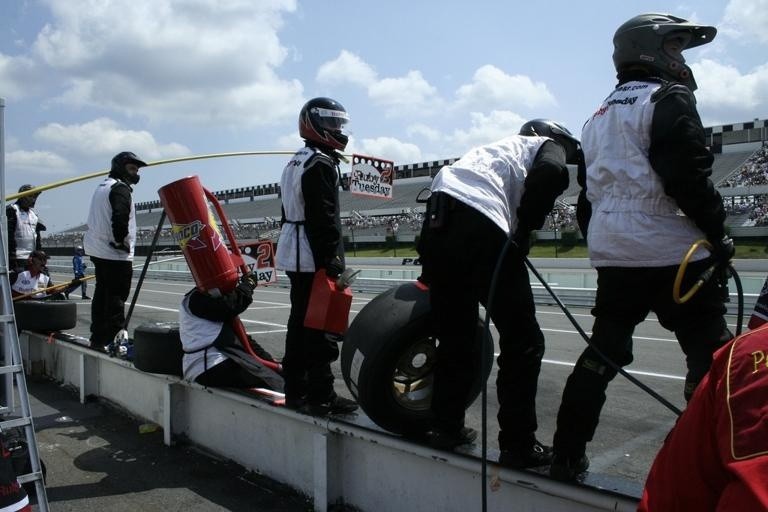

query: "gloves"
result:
(512, 220), (534, 255)
(241, 271), (258, 290)
(109, 241), (130, 253)
(326, 256), (344, 278)
(706, 231), (734, 268)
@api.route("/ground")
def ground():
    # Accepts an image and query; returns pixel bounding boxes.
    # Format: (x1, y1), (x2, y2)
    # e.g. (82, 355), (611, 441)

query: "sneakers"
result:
(64, 291), (69, 300)
(550, 452), (589, 482)
(285, 393), (311, 410)
(310, 397), (358, 416)
(423, 427), (478, 449)
(82, 296), (91, 299)
(499, 440), (553, 470)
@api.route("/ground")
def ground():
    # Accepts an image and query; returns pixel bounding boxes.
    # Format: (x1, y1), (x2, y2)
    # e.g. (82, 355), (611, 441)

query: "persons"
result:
(65, 246), (93, 298)
(639, 272), (768, 510)
(176, 274), (287, 393)
(90, 151), (146, 355)
(552, 54), (737, 480)
(137, 141), (577, 250)
(2, 185), (67, 305)
(718, 142), (767, 224)
(274, 98), (362, 415)
(419, 118), (582, 470)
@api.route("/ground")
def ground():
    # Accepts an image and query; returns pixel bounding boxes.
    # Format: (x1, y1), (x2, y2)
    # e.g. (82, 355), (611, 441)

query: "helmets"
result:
(18, 184), (41, 208)
(77, 245), (83, 253)
(26, 250), (50, 275)
(613, 13), (717, 92)
(299, 97), (348, 152)
(111, 152), (147, 185)
(518, 118), (582, 165)
